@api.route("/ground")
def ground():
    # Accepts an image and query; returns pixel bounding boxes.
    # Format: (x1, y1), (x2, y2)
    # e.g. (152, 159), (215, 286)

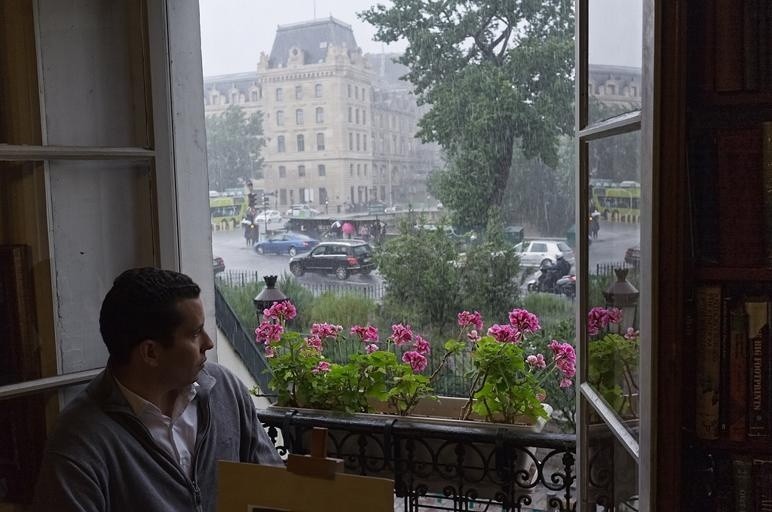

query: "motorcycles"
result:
(527, 265), (576, 298)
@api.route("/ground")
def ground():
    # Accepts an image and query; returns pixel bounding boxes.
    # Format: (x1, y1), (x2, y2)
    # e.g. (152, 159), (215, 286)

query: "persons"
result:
(545, 253), (571, 276)
(245, 224), (259, 246)
(333, 227), (349, 239)
(588, 215), (600, 239)
(359, 223), (386, 240)
(30, 267), (287, 512)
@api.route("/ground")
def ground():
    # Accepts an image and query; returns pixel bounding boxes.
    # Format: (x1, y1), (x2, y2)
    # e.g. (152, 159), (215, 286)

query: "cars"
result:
(493, 240), (576, 268)
(286, 206), (320, 218)
(254, 210), (282, 224)
(212, 254), (225, 278)
(289, 240), (378, 281)
(624, 243), (640, 267)
(254, 232), (319, 256)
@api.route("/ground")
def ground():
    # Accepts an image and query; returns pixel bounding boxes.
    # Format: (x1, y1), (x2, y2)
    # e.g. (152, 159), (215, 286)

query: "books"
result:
(681, 279), (772, 512)
(0, 244), (41, 381)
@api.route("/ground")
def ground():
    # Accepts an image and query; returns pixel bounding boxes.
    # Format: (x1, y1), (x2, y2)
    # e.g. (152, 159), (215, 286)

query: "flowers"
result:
(588, 305), (638, 423)
(347, 255), (360, 268)
(342, 223), (353, 234)
(252, 299), (574, 423)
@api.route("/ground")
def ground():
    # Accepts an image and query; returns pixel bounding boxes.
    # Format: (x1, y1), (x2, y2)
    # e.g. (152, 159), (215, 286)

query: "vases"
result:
(258, 388), (553, 503)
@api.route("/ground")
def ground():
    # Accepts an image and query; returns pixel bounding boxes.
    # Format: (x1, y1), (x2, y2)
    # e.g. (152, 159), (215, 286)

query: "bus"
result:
(210, 195), (247, 229)
(589, 186), (641, 220)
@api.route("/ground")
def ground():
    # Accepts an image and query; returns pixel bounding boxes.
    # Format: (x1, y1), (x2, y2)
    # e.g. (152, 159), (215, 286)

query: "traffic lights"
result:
(249, 193), (257, 207)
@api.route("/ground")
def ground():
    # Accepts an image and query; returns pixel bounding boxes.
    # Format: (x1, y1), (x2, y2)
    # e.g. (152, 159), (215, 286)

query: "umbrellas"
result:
(242, 220), (252, 225)
(330, 221), (355, 234)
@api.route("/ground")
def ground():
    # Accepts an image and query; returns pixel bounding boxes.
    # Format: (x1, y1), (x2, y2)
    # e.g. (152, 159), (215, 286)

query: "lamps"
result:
(604, 269), (641, 342)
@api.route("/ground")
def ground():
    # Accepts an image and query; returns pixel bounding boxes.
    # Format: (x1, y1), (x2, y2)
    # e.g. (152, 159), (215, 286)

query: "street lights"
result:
(602, 267), (639, 339)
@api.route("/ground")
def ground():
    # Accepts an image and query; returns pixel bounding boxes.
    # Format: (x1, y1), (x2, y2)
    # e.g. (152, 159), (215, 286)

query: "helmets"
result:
(555, 252), (564, 259)
